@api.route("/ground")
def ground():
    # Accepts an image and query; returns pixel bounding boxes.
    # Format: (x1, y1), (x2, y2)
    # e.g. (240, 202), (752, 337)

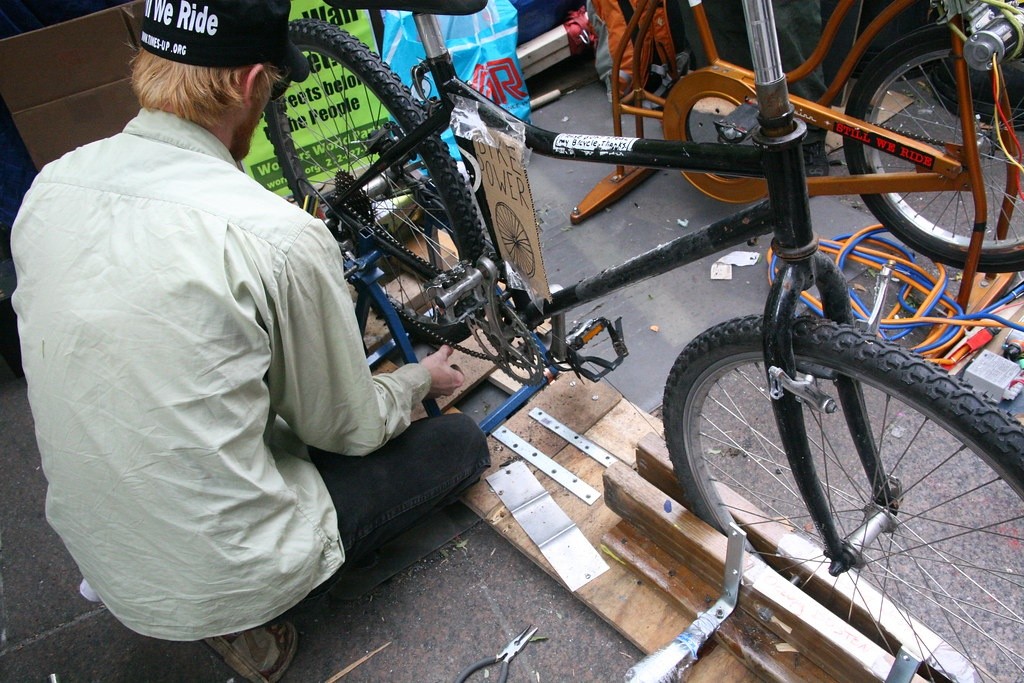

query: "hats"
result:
(141, 0), (309, 101)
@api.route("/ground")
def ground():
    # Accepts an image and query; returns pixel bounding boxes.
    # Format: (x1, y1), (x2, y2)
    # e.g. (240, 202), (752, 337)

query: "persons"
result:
(11, 0), (491, 683)
(680, 0), (830, 180)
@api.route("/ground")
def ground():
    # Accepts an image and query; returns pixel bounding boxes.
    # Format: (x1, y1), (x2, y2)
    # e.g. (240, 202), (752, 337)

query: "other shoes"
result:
(202, 617), (298, 683)
(802, 140), (828, 178)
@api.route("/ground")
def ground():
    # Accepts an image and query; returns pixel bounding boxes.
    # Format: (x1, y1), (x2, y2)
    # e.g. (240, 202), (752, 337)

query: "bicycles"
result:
(262, 0), (1024, 682)
